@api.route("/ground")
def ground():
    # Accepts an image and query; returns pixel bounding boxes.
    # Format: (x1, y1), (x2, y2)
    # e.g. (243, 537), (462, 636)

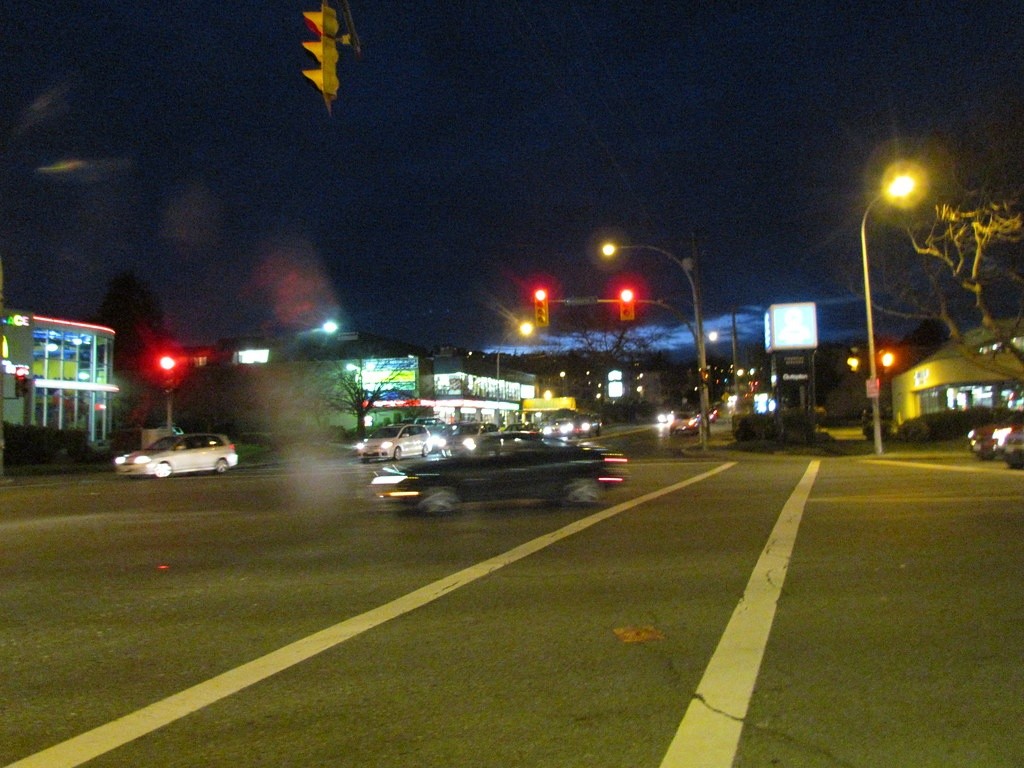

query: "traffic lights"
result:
(877, 348), (894, 376)
(619, 288), (634, 321)
(15, 367), (29, 398)
(302, 4), (339, 116)
(533, 289), (549, 327)
(847, 346), (861, 373)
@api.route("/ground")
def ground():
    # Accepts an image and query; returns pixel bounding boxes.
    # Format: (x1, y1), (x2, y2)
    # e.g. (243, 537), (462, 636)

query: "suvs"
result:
(357, 416), (604, 464)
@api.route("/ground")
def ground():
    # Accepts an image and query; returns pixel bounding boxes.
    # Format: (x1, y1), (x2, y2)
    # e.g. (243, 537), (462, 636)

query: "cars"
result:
(372, 432), (617, 516)
(659, 405), (719, 437)
(967, 411), (1024, 470)
(114, 433), (238, 479)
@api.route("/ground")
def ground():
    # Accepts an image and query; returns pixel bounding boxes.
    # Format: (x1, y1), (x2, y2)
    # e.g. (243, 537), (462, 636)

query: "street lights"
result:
(160, 355), (177, 435)
(497, 322), (533, 413)
(323, 320), (362, 437)
(860, 173), (915, 454)
(602, 243), (710, 449)
